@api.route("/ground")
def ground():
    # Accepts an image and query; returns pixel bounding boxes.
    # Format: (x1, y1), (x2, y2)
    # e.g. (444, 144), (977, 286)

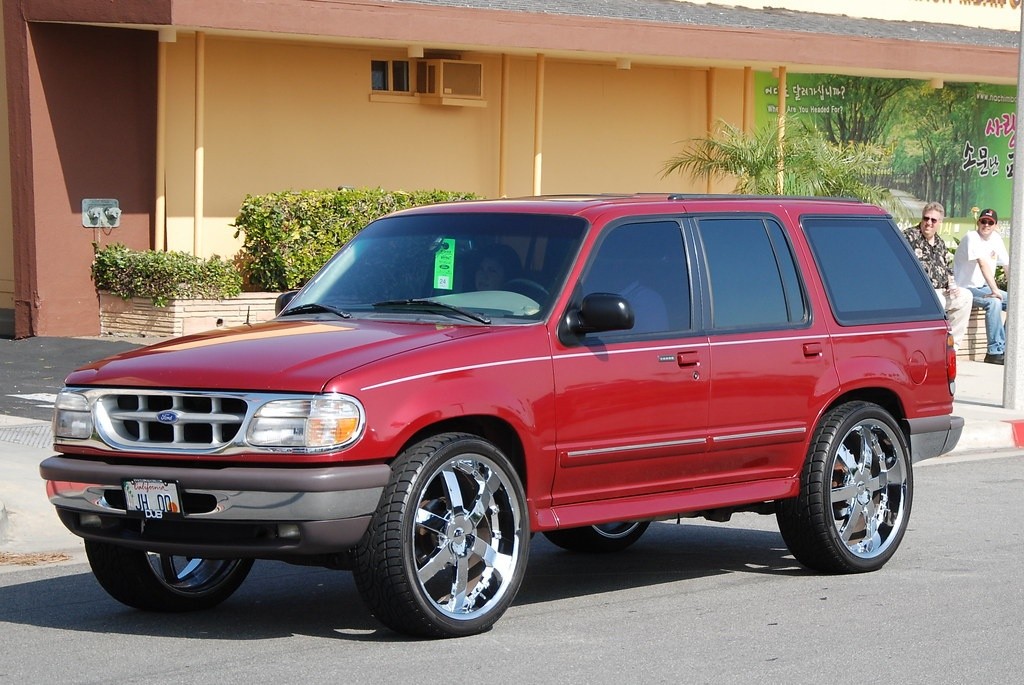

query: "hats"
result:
(979, 209), (997, 224)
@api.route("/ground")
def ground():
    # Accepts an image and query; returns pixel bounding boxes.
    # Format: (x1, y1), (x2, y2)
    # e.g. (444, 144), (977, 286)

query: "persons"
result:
(568, 238), (668, 338)
(462, 242), (524, 292)
(901, 201), (973, 353)
(953, 209), (1009, 365)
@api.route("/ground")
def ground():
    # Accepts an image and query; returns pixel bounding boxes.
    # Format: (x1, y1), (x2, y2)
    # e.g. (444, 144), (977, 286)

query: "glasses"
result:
(478, 265), (504, 276)
(923, 216), (938, 223)
(979, 220), (994, 225)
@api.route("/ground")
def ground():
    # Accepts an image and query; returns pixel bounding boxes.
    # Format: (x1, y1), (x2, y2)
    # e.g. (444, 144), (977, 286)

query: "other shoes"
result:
(984, 353), (1004, 365)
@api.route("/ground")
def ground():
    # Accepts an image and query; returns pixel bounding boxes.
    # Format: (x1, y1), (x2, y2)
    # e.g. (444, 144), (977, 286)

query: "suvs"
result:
(35, 191), (966, 643)
